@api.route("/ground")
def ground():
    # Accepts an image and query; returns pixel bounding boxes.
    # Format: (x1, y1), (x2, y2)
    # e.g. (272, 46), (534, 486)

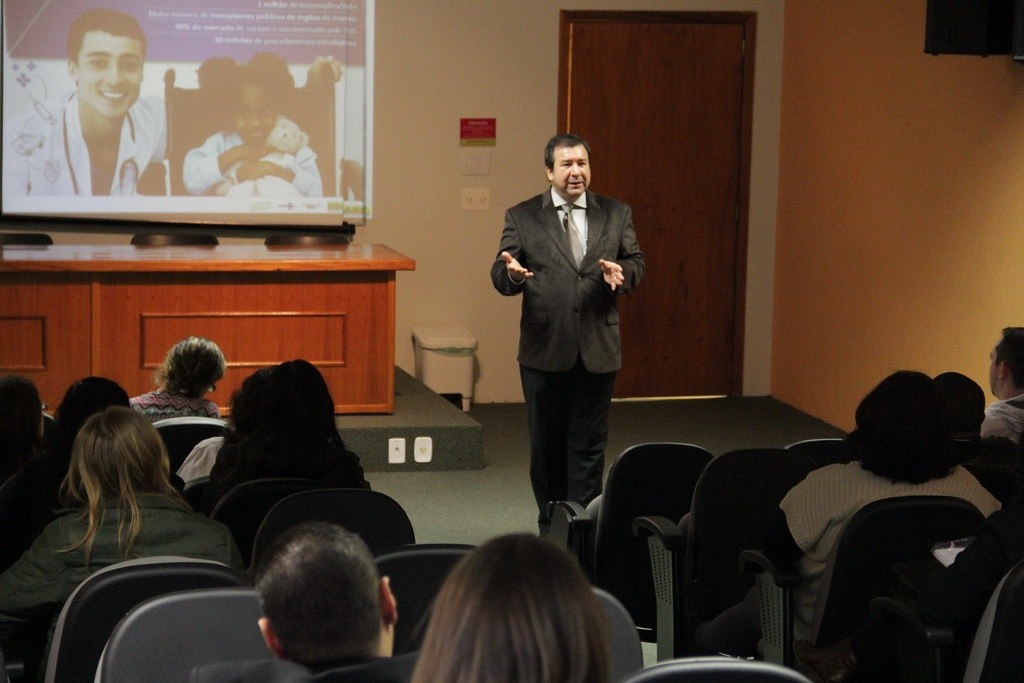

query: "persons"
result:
(694, 327), (1024, 683)
(409, 533), (613, 683)
(181, 54), (323, 198)
(0, 335), (372, 683)
(489, 133), (647, 536)
(188, 520), (421, 682)
(4, 8), (168, 197)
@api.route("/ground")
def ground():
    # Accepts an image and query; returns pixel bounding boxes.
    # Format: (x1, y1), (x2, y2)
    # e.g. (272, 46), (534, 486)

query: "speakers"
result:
(923, 0), (1024, 59)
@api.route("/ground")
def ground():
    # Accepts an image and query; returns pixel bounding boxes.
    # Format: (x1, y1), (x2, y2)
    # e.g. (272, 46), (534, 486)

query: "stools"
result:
(412, 327), (477, 412)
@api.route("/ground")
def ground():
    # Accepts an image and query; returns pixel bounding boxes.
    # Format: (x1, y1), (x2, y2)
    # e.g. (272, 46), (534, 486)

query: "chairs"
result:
(265, 232), (349, 248)
(0, 415), (1024, 683)
(131, 232), (218, 246)
(0, 232), (52, 244)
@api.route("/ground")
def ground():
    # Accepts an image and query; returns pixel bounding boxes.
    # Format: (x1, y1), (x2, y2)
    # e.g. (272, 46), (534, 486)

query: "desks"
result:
(0, 242), (414, 415)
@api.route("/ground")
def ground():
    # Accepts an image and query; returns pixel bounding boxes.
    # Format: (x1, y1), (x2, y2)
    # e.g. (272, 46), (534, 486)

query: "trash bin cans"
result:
(413, 325), (477, 412)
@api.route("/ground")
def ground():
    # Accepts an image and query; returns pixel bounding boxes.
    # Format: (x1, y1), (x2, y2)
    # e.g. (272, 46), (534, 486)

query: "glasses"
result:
(41, 402), (49, 411)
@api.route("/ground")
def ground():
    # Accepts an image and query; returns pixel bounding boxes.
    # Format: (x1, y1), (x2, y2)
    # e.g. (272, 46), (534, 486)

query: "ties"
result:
(561, 202), (585, 269)
